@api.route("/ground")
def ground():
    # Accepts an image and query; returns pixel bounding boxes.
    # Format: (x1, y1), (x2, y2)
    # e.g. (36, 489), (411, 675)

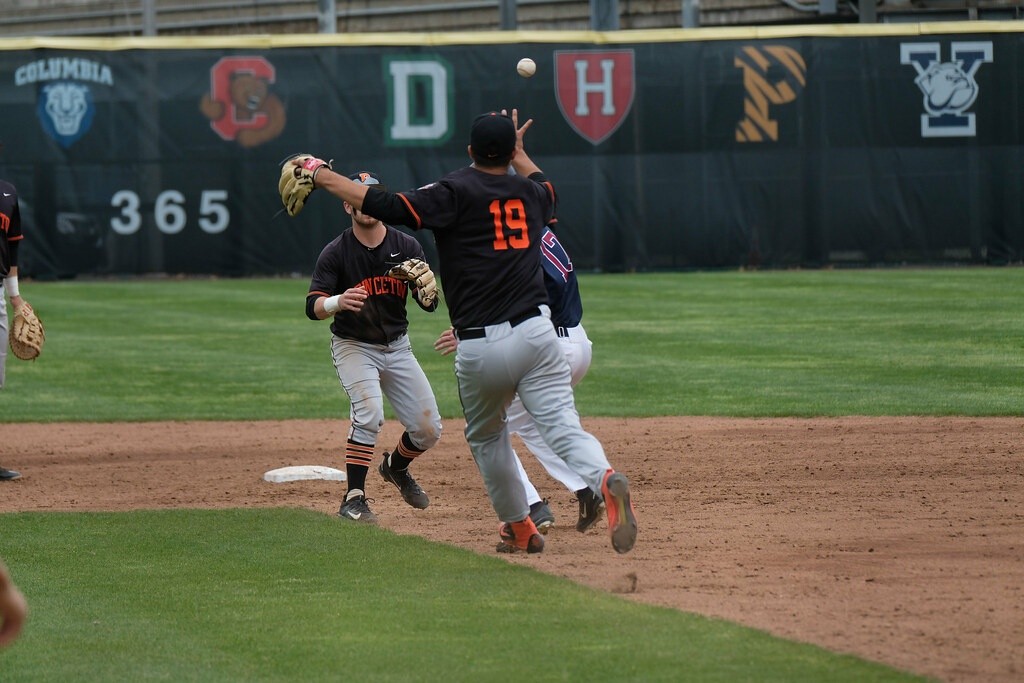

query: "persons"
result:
(0, 560), (29, 645)
(306, 171), (444, 526)
(433, 163), (607, 537)
(278, 108), (636, 555)
(0, 180), (25, 480)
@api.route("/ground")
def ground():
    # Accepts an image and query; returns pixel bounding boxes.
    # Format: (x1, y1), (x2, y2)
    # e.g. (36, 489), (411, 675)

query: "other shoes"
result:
(0, 467), (22, 482)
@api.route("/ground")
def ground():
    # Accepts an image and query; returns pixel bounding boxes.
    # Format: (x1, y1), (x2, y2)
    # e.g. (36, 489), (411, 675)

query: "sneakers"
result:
(378, 451), (430, 508)
(337, 489), (377, 522)
(528, 497), (555, 534)
(570, 486), (606, 532)
(496, 516), (544, 552)
(601, 468), (638, 554)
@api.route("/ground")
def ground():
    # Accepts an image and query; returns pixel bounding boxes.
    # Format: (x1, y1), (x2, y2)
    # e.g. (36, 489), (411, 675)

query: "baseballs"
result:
(517, 57), (537, 78)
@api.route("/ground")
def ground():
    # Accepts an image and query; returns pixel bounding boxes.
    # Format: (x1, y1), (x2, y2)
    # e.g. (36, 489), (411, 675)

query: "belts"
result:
(457, 306), (542, 340)
(556, 327), (569, 338)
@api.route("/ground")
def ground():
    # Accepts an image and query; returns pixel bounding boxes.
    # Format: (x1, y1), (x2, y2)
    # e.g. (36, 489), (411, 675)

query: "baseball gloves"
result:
(8, 300), (47, 364)
(271, 151), (335, 219)
(382, 255), (445, 313)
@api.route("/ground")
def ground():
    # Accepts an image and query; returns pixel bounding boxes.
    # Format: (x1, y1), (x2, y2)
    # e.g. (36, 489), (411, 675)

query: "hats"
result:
(348, 171), (381, 185)
(471, 111), (516, 157)
